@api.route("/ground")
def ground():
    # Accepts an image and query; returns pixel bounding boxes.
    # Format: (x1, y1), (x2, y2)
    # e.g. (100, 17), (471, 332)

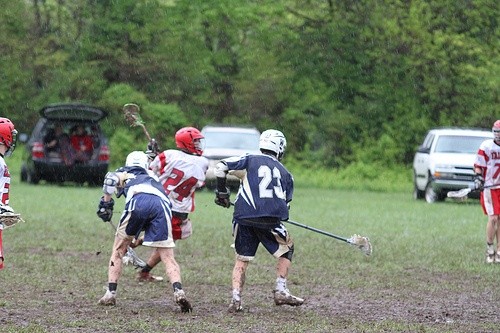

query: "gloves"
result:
(129, 238), (142, 247)
(98, 196), (114, 223)
(473, 175), (484, 190)
(215, 187), (231, 207)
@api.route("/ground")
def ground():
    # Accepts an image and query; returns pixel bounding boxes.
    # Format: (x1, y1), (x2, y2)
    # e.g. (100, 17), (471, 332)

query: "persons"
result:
(97, 151), (193, 314)
(0, 117), (23, 264)
(45, 121), (71, 158)
(214, 128), (304, 312)
(130, 127), (209, 286)
(70, 123), (94, 159)
(472, 119), (500, 263)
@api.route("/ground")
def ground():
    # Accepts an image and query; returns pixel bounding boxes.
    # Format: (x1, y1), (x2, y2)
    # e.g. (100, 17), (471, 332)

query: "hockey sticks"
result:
(230, 201), (372, 257)
(447, 184), (500, 199)
(123, 103), (153, 143)
(100, 207), (146, 268)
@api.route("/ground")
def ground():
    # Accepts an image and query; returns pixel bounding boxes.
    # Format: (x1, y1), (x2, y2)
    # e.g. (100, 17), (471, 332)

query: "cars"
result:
(410, 126), (495, 204)
(194, 124), (264, 192)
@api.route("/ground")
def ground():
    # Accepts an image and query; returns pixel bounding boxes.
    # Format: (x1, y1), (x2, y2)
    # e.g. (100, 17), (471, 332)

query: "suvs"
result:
(18, 103), (111, 188)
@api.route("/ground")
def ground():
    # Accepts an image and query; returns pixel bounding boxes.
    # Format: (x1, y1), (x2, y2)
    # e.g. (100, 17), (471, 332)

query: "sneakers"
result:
(494, 252), (500, 263)
(97, 287), (117, 305)
(273, 289), (304, 306)
(135, 272), (164, 285)
(486, 248), (495, 264)
(174, 288), (192, 311)
(229, 299), (243, 313)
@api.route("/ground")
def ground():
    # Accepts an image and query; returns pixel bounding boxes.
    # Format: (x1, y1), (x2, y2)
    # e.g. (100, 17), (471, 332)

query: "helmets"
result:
(0, 116), (17, 152)
(492, 120), (500, 138)
(259, 129), (287, 161)
(175, 128), (204, 156)
(126, 150), (149, 170)
